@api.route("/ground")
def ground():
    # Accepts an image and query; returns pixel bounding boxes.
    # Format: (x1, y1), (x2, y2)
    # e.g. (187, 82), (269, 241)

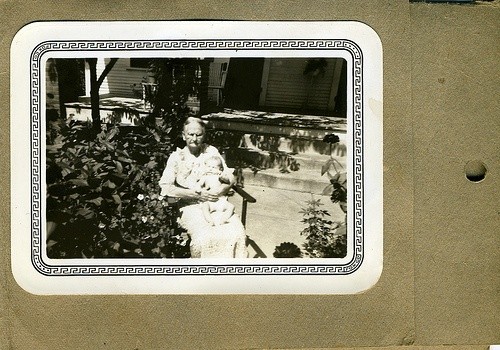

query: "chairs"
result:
(166, 176), (257, 257)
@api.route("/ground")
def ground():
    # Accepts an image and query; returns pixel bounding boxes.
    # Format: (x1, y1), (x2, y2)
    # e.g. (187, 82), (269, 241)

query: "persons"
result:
(158, 116), (250, 258)
(191, 156), (236, 227)
